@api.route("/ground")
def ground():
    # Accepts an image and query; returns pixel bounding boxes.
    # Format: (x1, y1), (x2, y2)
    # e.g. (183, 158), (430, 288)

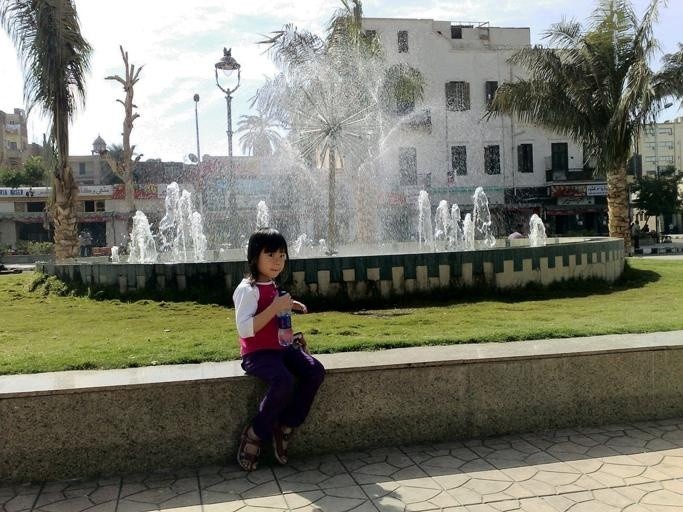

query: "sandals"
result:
(271, 422), (294, 465)
(235, 423), (261, 472)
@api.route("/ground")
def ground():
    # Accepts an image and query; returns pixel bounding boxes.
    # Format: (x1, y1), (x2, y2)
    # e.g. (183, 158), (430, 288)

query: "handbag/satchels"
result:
(291, 331), (310, 356)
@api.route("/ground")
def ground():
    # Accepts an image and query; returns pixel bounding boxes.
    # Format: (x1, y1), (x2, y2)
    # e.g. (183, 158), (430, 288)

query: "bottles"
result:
(277, 291), (293, 346)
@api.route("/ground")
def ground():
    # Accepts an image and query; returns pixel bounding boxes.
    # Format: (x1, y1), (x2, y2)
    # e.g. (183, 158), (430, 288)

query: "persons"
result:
(631, 220), (640, 248)
(234, 227), (325, 472)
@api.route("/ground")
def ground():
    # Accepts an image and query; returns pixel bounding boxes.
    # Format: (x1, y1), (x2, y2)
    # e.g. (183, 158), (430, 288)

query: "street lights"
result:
(653, 102), (673, 243)
(215, 46), (241, 250)
(188, 93), (210, 249)
